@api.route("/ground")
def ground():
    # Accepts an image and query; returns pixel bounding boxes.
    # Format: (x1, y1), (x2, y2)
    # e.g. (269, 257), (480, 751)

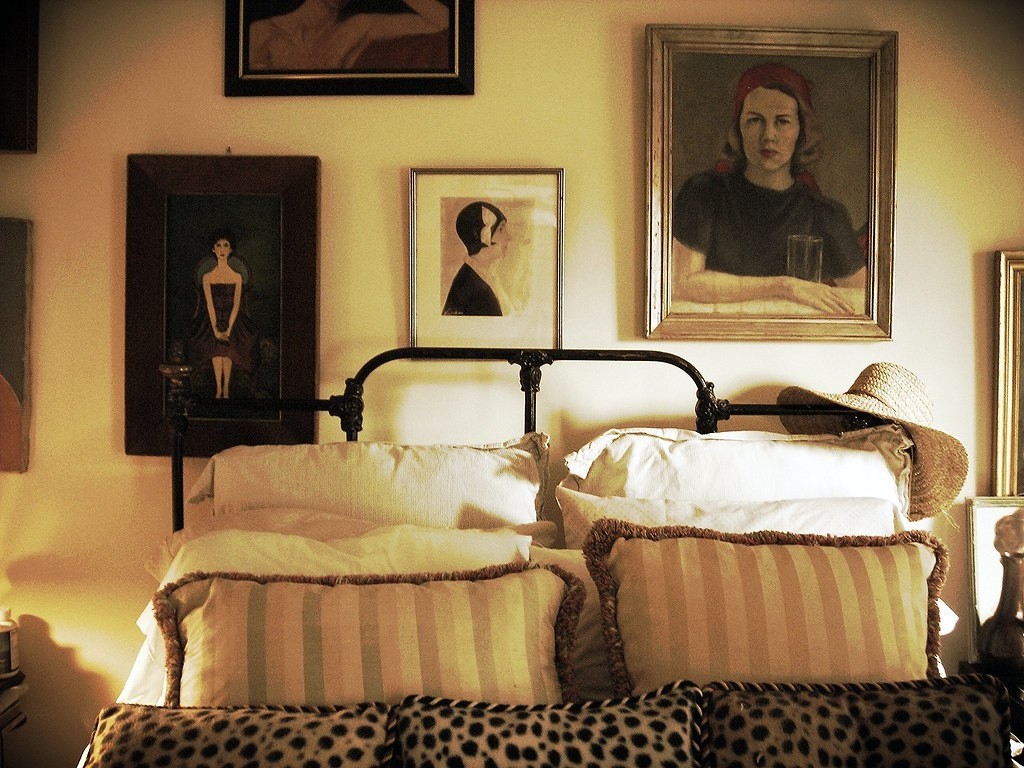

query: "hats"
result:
(776, 361), (968, 522)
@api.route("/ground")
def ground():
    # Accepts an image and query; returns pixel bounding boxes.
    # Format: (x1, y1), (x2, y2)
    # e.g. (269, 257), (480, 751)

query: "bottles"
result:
(0, 608), (19, 678)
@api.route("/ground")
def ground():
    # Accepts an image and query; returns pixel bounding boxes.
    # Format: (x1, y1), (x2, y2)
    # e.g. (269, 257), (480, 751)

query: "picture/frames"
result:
(0, 0), (40, 155)
(409, 165), (567, 353)
(223, 0), (477, 96)
(124, 152), (324, 460)
(643, 21), (903, 343)
(0, 216), (35, 474)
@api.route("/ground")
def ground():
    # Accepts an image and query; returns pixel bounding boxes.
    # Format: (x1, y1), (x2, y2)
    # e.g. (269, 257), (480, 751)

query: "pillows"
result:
(556, 426), (919, 527)
(150, 558), (593, 710)
(183, 430), (551, 531)
(698, 671), (1016, 768)
(78, 697), (399, 768)
(394, 677), (713, 768)
(577, 518), (954, 695)
